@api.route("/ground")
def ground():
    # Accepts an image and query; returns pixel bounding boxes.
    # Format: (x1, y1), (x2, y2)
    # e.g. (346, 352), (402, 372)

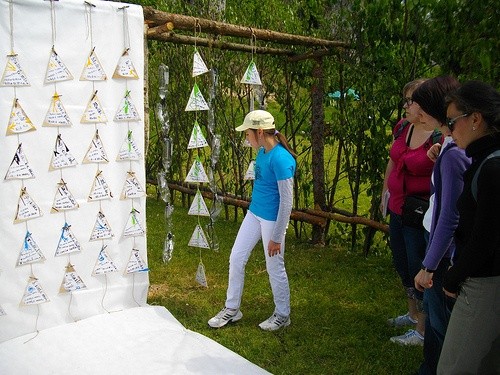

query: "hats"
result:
(234, 110), (275, 131)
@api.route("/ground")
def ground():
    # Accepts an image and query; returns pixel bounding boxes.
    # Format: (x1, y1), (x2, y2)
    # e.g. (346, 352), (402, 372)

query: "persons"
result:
(378, 77), (444, 348)
(207, 109), (299, 332)
(435, 80), (500, 375)
(411, 72), (473, 375)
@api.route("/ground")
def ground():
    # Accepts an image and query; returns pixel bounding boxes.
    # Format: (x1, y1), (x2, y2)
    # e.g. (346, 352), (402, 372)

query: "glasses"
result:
(402, 98), (414, 106)
(446, 111), (474, 131)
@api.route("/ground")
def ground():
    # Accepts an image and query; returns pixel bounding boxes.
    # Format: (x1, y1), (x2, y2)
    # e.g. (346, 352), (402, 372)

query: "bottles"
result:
(208, 69), (217, 99)
(206, 172), (223, 219)
(252, 85), (266, 108)
(163, 205), (174, 268)
(157, 63), (170, 103)
(155, 171), (171, 205)
(207, 102), (215, 133)
(163, 139), (172, 174)
(211, 135), (221, 171)
(204, 223), (220, 254)
(154, 103), (171, 138)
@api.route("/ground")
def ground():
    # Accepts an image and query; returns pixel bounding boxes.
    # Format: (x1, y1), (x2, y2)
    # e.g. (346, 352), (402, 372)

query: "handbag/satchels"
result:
(401, 194), (431, 221)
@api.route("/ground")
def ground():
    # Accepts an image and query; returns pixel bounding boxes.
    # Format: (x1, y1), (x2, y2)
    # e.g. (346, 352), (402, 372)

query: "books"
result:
(381, 188), (391, 219)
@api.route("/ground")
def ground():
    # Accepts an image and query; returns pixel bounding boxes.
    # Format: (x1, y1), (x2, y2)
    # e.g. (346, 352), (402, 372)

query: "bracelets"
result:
(421, 265), (435, 273)
(272, 241), (278, 245)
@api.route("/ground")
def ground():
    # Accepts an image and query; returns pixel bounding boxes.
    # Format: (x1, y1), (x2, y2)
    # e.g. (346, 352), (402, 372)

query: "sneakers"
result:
(259, 312), (292, 332)
(388, 311), (418, 327)
(208, 306), (243, 327)
(390, 329), (426, 347)
